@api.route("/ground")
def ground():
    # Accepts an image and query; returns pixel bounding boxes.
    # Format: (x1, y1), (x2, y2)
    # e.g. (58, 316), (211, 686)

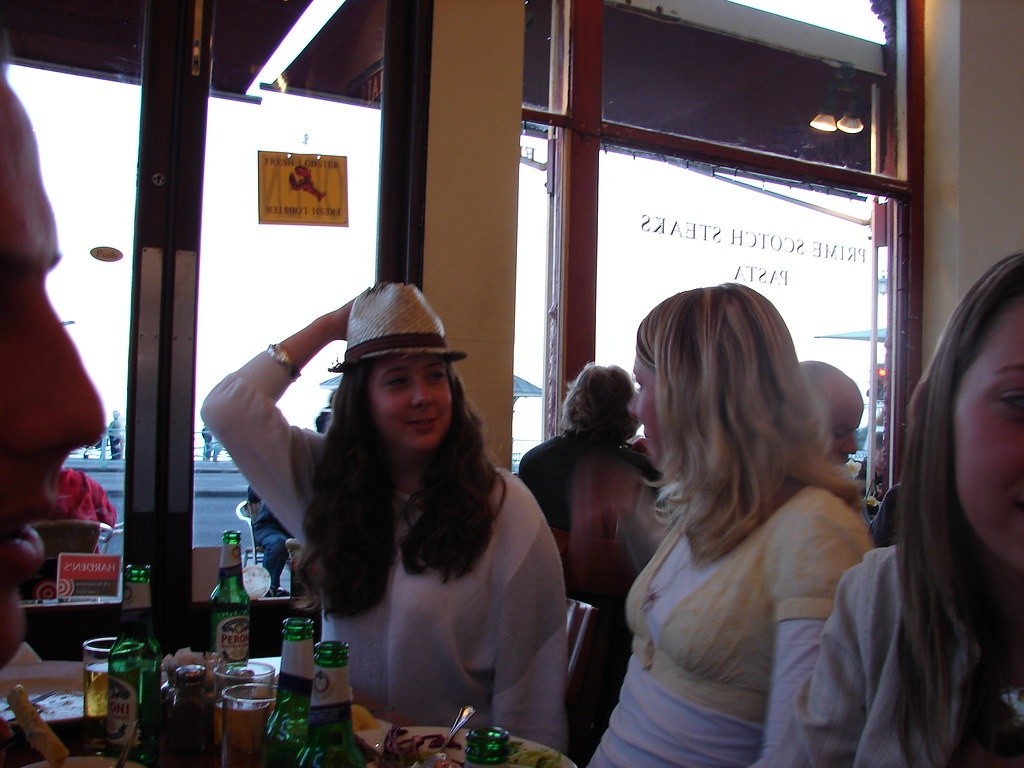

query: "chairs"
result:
(235, 501), (290, 567)
(33, 520), (113, 596)
(558, 596), (603, 692)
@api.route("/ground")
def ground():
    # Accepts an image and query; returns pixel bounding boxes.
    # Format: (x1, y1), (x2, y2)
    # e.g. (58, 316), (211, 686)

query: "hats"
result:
(327, 280), (468, 374)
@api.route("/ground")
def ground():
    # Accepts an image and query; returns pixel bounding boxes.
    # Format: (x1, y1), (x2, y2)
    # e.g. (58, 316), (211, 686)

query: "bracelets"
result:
(267, 344), (301, 381)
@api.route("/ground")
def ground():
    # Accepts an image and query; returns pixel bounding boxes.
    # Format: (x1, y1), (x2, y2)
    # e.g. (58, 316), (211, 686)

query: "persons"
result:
(17, 465), (117, 599)
(199, 280), (569, 753)
(512, 252), (1024, 768)
(0, 66), (106, 678)
(247, 483), (294, 597)
(202, 424), (221, 462)
(315, 411), (332, 433)
(106, 410), (124, 460)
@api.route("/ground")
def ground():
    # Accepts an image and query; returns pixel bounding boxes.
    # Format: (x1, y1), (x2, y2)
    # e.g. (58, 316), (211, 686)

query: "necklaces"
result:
(639, 540), (694, 612)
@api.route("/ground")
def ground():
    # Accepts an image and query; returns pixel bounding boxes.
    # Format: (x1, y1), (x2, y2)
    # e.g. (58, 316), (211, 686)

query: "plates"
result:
(354, 725), (579, 768)
(21, 755), (147, 768)
(0, 661), (84, 725)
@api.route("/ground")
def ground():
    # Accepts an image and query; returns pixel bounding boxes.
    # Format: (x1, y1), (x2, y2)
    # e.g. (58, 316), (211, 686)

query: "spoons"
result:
(420, 704), (475, 767)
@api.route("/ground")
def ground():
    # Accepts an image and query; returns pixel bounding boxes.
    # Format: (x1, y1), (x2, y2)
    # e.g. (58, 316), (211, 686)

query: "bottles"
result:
(289, 640), (366, 768)
(463, 725), (510, 768)
(260, 617), (316, 768)
(167, 663), (212, 757)
(208, 530), (250, 666)
(106, 563), (163, 768)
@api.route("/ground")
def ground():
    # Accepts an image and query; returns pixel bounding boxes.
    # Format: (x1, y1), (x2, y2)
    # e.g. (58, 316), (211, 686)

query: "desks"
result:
(0, 656), (416, 768)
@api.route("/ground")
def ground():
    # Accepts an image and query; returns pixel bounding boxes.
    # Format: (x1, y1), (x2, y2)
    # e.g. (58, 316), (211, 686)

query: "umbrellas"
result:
(812, 328), (887, 344)
(319, 373), (542, 408)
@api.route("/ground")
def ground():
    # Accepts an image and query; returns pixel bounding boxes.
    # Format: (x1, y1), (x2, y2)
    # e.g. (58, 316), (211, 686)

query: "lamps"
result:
(811, 61), (846, 135)
(837, 70), (872, 133)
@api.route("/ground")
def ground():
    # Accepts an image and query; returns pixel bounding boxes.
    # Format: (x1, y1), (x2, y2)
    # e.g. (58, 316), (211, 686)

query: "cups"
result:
(212, 661), (275, 768)
(219, 683), (278, 768)
(82, 636), (117, 752)
